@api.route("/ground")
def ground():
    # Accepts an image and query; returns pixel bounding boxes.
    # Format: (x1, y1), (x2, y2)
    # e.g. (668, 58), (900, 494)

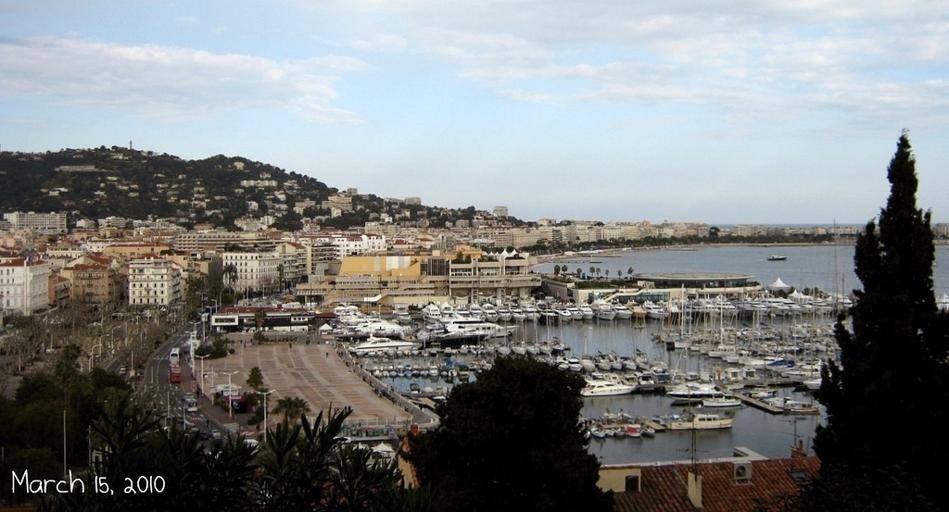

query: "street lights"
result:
(88, 319), (135, 373)
(223, 371), (240, 417)
(184, 321), (210, 396)
(256, 389), (276, 443)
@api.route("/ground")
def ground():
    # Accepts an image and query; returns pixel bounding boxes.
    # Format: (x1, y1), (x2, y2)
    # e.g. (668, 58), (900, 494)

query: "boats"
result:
(768, 255), (787, 260)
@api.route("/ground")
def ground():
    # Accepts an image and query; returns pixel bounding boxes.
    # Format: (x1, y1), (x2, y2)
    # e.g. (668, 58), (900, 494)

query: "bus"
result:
(185, 399), (199, 412)
(170, 348), (181, 365)
(170, 364), (182, 383)
